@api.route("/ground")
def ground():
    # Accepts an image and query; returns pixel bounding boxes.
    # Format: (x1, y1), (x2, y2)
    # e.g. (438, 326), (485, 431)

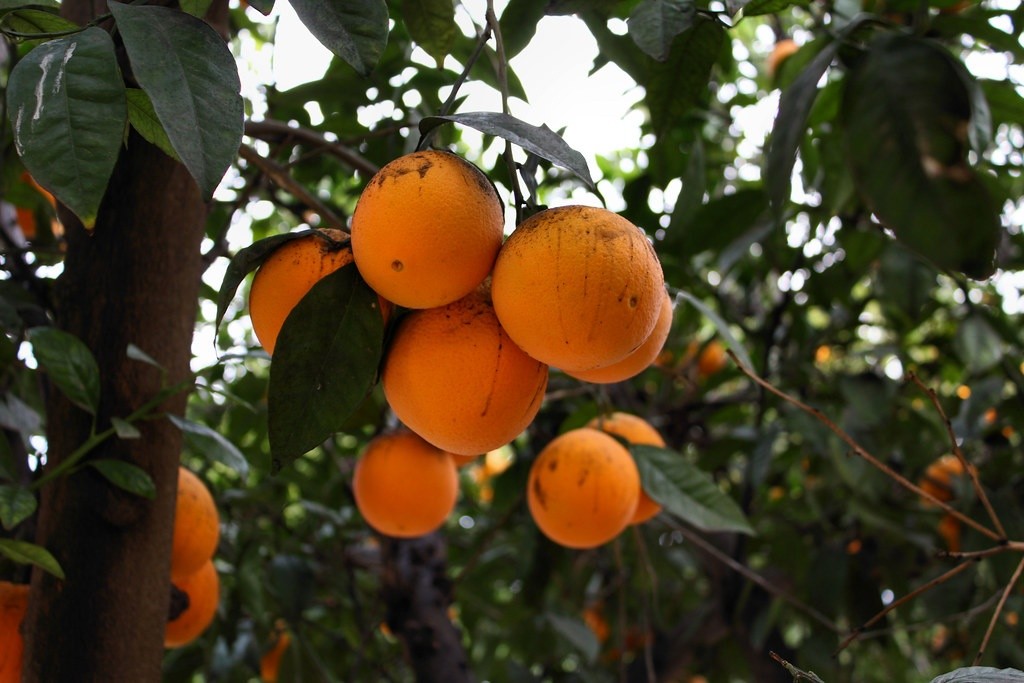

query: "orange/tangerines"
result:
(0, 0), (1022, 682)
